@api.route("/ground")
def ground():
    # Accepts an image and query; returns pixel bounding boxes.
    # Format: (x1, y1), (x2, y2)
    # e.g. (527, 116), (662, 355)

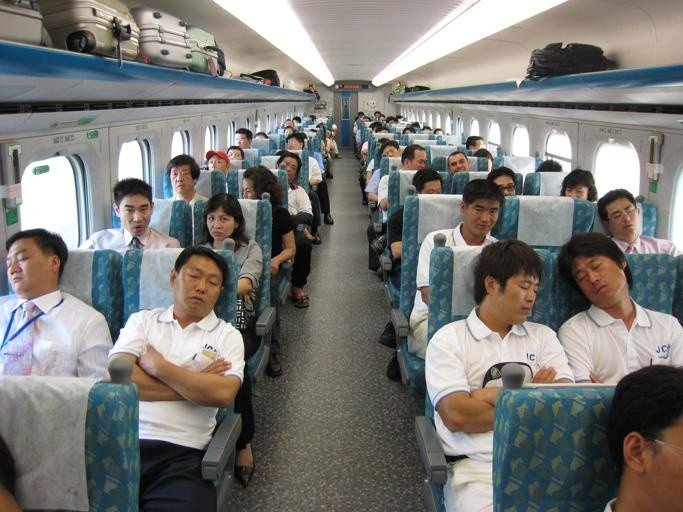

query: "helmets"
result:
(206, 150), (231, 169)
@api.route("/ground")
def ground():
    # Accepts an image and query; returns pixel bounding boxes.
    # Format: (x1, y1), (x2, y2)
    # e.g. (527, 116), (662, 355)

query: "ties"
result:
(2, 303), (37, 376)
(130, 237), (140, 251)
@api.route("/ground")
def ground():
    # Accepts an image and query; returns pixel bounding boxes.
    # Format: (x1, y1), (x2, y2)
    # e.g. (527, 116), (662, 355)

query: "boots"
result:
(359, 178), (368, 205)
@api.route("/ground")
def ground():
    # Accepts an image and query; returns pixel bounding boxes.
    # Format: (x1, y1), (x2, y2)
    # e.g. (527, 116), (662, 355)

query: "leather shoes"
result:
(311, 232), (321, 245)
(234, 441), (256, 488)
(324, 213), (333, 225)
(290, 286), (310, 308)
(266, 352), (282, 376)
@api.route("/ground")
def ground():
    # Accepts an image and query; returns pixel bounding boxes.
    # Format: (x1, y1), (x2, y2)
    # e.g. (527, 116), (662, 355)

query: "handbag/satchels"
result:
(240, 73), (264, 85)
(236, 300), (251, 329)
(252, 70), (280, 87)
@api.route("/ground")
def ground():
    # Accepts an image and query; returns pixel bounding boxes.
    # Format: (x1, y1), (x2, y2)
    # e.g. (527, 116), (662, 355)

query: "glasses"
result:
(500, 185), (515, 191)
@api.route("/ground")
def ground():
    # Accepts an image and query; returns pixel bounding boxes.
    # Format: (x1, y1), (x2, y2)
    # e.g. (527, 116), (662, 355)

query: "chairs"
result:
(61, 238), (242, 509)
(110, 113), (336, 385)
(1, 356), (140, 511)
(412, 233), (682, 511)
(353, 111), (659, 389)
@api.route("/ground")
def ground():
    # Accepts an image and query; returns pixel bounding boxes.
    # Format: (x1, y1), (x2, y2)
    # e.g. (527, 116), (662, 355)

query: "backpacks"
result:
(524, 42), (615, 81)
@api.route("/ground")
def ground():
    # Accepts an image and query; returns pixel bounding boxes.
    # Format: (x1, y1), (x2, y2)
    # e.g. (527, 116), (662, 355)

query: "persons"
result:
(603, 365), (682, 511)
(352, 111), (683, 382)
(109, 247), (245, 509)
(0, 436), (23, 510)
(425, 239), (574, 510)
(0, 229), (113, 379)
(81, 110), (343, 484)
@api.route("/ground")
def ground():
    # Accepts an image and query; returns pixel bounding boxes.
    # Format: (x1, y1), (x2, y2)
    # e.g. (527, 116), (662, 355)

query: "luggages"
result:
(1, 0), (226, 77)
